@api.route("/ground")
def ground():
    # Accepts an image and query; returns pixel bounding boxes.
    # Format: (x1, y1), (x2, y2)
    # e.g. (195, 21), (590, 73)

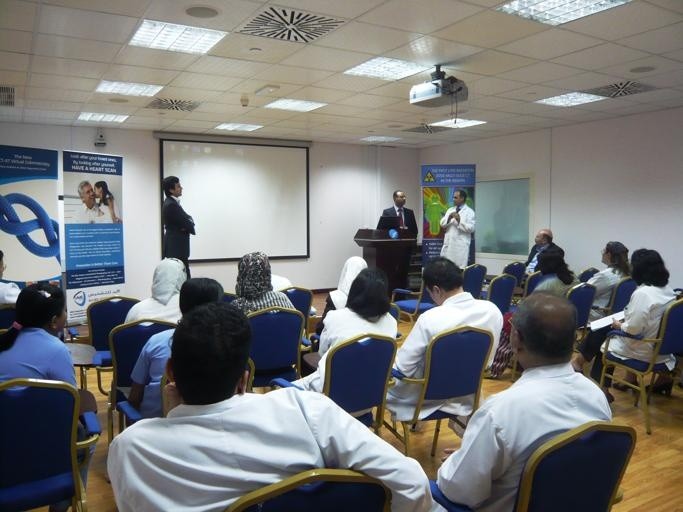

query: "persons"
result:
(488, 229), (676, 400)
(69, 181), (98, 224)
(433, 290), (611, 512)
(382, 257), (503, 424)
(439, 190), (476, 272)
(1, 250), (87, 512)
(377, 190), (418, 300)
(125, 253), (397, 420)
(161, 176), (196, 278)
(93, 181), (122, 224)
(107, 302), (434, 512)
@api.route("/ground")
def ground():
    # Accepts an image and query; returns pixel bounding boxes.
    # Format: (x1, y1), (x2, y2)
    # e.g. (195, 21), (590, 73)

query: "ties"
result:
(397, 207), (402, 227)
(456, 205), (459, 214)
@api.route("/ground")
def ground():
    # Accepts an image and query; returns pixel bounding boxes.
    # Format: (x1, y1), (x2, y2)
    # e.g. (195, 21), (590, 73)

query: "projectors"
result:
(410, 76), (468, 108)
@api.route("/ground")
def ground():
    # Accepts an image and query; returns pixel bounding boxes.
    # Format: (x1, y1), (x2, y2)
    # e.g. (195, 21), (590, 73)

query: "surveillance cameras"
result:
(94, 133), (106, 146)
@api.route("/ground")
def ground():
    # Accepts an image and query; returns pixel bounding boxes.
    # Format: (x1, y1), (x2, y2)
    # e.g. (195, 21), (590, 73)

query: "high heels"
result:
(613, 380), (638, 393)
(645, 379), (672, 396)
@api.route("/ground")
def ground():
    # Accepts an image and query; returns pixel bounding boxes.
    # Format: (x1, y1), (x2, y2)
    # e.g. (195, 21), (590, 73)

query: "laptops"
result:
(376, 216), (401, 229)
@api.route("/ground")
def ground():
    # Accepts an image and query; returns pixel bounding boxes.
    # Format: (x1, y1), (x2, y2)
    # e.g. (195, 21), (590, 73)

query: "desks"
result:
(66, 342), (98, 367)
(76, 388), (97, 417)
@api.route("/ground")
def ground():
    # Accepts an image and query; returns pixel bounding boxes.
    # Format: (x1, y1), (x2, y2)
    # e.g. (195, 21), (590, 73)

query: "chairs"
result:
(226, 469), (391, 511)
(86, 295), (141, 350)
(1, 376), (99, 511)
(108, 319), (178, 446)
(0, 303), (16, 329)
(244, 305), (305, 394)
(515, 419), (636, 512)
(117, 356), (255, 440)
(268, 333), (398, 440)
(279, 285), (313, 345)
